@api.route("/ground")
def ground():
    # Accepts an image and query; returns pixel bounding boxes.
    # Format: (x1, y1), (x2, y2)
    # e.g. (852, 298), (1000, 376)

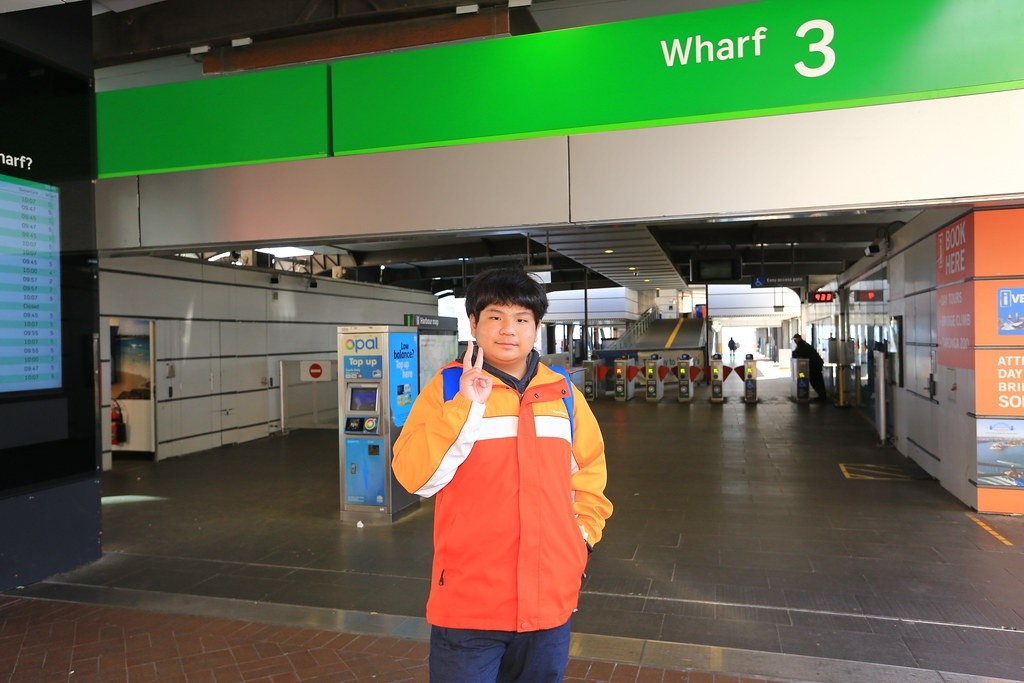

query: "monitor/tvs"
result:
(699, 258), (736, 281)
(350, 388), (377, 411)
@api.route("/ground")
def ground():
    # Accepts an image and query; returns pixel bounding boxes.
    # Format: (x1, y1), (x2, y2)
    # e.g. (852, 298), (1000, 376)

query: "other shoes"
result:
(814, 396), (827, 401)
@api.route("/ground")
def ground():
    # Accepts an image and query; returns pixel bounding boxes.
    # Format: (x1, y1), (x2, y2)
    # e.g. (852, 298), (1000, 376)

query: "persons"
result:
(791, 334), (827, 401)
(391, 265), (614, 683)
(728, 337), (736, 355)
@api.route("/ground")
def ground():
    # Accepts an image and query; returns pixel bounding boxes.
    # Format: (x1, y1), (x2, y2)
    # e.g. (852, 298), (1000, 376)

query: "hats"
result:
(792, 334), (801, 339)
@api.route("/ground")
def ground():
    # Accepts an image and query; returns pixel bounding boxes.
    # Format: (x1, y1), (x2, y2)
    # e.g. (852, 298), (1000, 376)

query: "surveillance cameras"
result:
(271, 273), (281, 283)
(309, 277), (317, 288)
(864, 244), (881, 256)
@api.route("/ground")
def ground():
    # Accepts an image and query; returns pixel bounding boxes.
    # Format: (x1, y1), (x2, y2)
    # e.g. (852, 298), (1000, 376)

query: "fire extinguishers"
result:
(111, 397), (126, 445)
